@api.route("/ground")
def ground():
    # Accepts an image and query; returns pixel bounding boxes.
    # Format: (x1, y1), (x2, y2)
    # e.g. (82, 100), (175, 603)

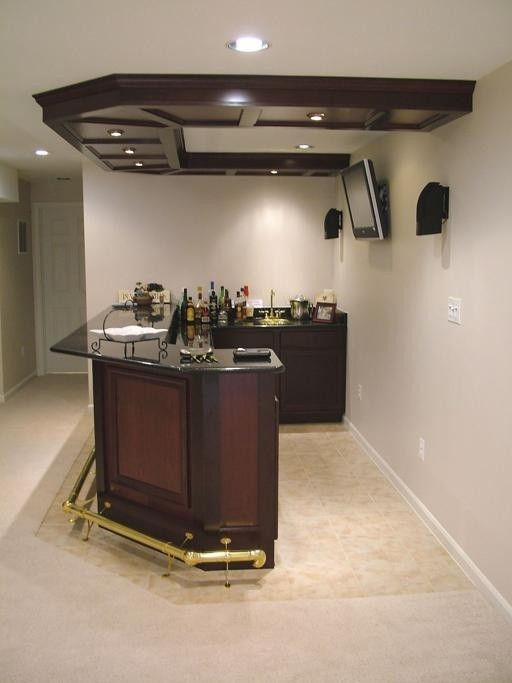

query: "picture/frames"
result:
(312, 303), (337, 324)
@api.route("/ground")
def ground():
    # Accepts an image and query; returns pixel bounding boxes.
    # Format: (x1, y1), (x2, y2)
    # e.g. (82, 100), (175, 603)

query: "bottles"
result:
(181, 325), (211, 349)
(181, 281), (254, 324)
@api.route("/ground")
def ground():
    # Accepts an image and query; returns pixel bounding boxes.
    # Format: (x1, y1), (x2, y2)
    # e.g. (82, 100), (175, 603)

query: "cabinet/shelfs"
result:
(278, 328), (346, 425)
(217, 328), (276, 348)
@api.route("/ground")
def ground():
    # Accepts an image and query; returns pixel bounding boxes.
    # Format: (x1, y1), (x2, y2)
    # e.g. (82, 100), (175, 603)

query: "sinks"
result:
(252, 317), (293, 326)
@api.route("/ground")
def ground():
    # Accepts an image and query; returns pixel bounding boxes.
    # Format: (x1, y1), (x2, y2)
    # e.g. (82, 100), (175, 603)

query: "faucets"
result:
(270, 288), (276, 318)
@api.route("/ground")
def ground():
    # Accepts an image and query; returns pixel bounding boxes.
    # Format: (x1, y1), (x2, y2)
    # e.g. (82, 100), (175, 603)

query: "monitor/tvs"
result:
(338, 159), (388, 240)
